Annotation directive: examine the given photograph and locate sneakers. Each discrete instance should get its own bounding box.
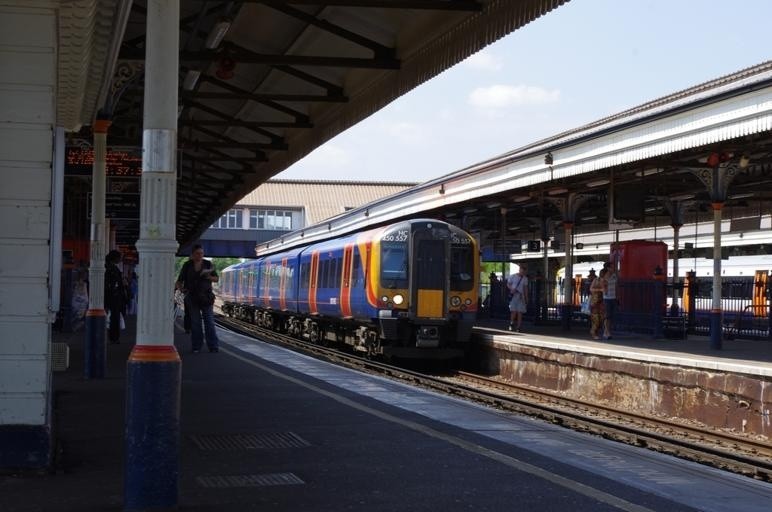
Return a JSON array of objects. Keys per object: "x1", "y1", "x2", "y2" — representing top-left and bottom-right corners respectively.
[
  {"x1": 516, "y1": 328, "x2": 521, "y2": 332},
  {"x1": 209, "y1": 348, "x2": 218, "y2": 353},
  {"x1": 508, "y1": 324, "x2": 512, "y2": 331},
  {"x1": 192, "y1": 349, "x2": 200, "y2": 353},
  {"x1": 592, "y1": 334, "x2": 612, "y2": 340}
]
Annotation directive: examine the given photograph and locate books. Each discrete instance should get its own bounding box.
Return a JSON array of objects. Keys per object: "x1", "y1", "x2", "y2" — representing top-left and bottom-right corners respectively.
[{"x1": 200, "y1": 269, "x2": 213, "y2": 277}]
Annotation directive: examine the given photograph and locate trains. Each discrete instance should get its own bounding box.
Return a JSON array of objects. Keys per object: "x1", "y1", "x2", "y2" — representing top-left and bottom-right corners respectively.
[
  {"x1": 554, "y1": 255, "x2": 771, "y2": 330},
  {"x1": 217, "y1": 218, "x2": 481, "y2": 374}
]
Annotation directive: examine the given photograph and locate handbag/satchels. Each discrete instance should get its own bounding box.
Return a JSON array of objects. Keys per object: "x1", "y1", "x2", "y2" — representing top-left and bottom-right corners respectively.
[
  {"x1": 590, "y1": 292, "x2": 602, "y2": 307},
  {"x1": 192, "y1": 287, "x2": 214, "y2": 306},
  {"x1": 508, "y1": 292, "x2": 513, "y2": 301},
  {"x1": 105, "y1": 312, "x2": 125, "y2": 329}
]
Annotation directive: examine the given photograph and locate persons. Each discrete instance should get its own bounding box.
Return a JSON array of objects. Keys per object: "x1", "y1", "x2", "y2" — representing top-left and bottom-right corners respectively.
[
  {"x1": 175, "y1": 244, "x2": 220, "y2": 355},
  {"x1": 589, "y1": 268, "x2": 610, "y2": 340},
  {"x1": 126, "y1": 271, "x2": 138, "y2": 315},
  {"x1": 506, "y1": 266, "x2": 529, "y2": 332},
  {"x1": 600, "y1": 262, "x2": 622, "y2": 339},
  {"x1": 104, "y1": 250, "x2": 126, "y2": 344}
]
